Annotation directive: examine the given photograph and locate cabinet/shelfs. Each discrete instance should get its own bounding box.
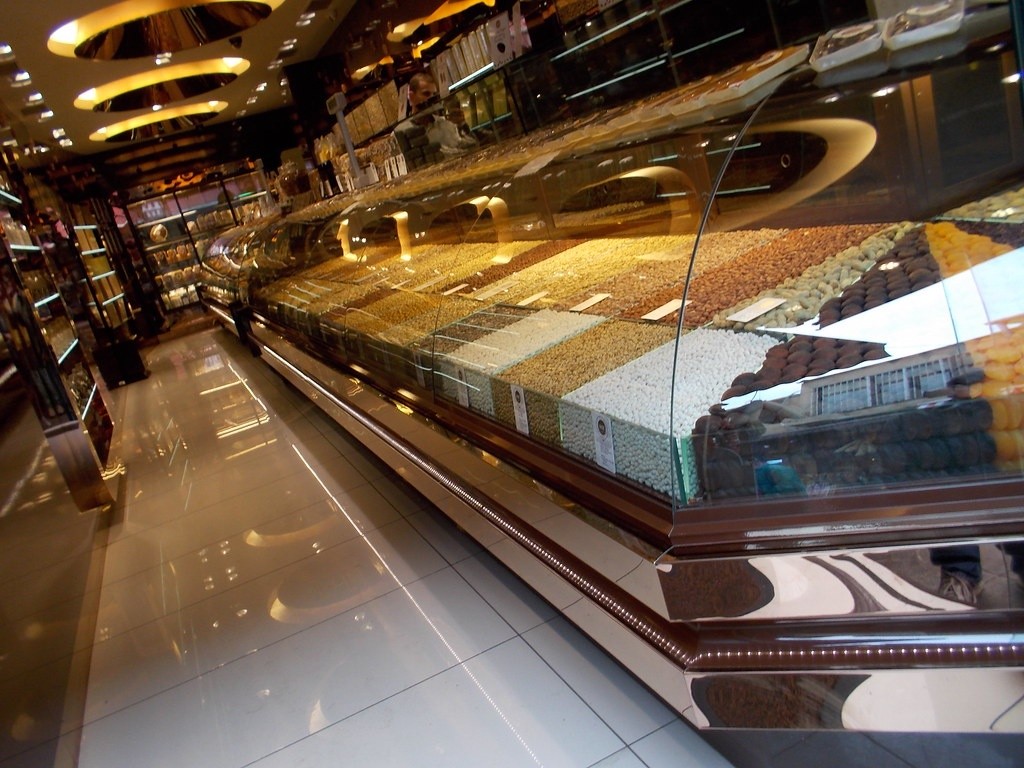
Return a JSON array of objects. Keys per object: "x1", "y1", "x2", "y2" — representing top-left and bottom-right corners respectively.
[{"x1": 0, "y1": 149, "x2": 277, "y2": 513}]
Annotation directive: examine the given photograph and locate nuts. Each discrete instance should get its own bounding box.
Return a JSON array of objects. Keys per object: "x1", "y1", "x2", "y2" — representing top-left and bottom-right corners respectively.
[{"x1": 573, "y1": 221, "x2": 917, "y2": 329}]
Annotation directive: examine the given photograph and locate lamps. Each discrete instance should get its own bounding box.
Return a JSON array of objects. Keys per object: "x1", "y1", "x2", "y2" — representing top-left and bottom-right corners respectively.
[
  {"x1": 73, "y1": 57, "x2": 251, "y2": 113},
  {"x1": 88, "y1": 101, "x2": 228, "y2": 143},
  {"x1": 46, "y1": 0, "x2": 285, "y2": 62}
]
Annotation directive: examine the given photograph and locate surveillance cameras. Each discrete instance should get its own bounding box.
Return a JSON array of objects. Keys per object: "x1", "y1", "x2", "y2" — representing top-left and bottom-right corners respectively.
[{"x1": 229, "y1": 36, "x2": 242, "y2": 48}]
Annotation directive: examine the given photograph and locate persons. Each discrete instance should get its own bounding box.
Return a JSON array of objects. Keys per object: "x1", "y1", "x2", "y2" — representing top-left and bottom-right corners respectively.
[
  {"x1": 391, "y1": 75, "x2": 478, "y2": 156},
  {"x1": 930, "y1": 541, "x2": 1024, "y2": 607}
]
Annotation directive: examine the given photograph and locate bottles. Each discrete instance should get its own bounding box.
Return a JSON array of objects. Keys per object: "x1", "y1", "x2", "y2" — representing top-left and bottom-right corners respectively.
[
  {"x1": 65, "y1": 362, "x2": 94, "y2": 414},
  {"x1": 1, "y1": 216, "x2": 33, "y2": 246},
  {"x1": 315, "y1": 150, "x2": 346, "y2": 200},
  {"x1": 40, "y1": 315, "x2": 75, "y2": 360},
  {"x1": 20, "y1": 269, "x2": 56, "y2": 302}
]
